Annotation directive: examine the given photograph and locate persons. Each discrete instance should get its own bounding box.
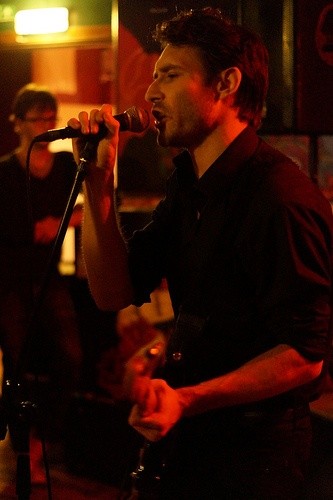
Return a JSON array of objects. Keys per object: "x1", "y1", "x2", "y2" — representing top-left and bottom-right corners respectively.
[
  {"x1": 67, "y1": 6, "x2": 333, "y2": 500},
  {"x1": 0, "y1": 91, "x2": 87, "y2": 408}
]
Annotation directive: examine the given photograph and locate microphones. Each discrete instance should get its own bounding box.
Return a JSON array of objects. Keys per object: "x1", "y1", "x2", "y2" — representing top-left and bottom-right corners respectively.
[{"x1": 33, "y1": 106, "x2": 149, "y2": 142}]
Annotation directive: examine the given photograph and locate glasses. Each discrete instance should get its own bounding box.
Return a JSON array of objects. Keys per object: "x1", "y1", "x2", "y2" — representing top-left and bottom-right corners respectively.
[{"x1": 23, "y1": 116, "x2": 58, "y2": 127}]
